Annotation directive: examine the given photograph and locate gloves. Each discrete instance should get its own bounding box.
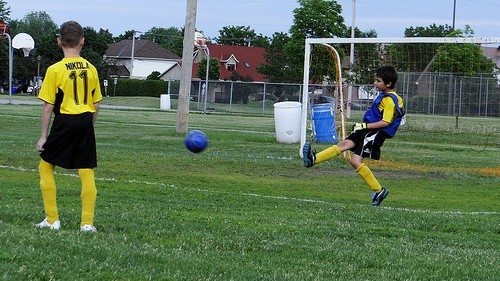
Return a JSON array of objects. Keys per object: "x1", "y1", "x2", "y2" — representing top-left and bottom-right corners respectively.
[{"x1": 352, "y1": 122, "x2": 367, "y2": 133}]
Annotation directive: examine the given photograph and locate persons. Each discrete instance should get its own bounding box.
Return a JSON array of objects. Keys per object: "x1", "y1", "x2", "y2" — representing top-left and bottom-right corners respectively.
[
  {"x1": 34, "y1": 21, "x2": 102, "y2": 231},
  {"x1": 303, "y1": 66, "x2": 406, "y2": 206}
]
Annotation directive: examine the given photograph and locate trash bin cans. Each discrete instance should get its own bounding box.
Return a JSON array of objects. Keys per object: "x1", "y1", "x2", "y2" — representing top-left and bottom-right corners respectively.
[
  {"x1": 273, "y1": 101, "x2": 302, "y2": 143},
  {"x1": 310, "y1": 103, "x2": 338, "y2": 144},
  {"x1": 160, "y1": 94, "x2": 170, "y2": 110}
]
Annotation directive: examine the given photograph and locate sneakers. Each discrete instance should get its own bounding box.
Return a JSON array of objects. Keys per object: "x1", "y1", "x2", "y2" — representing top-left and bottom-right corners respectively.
[
  {"x1": 80, "y1": 224, "x2": 97, "y2": 233},
  {"x1": 35, "y1": 217, "x2": 60, "y2": 230},
  {"x1": 371, "y1": 187, "x2": 389, "y2": 206},
  {"x1": 303, "y1": 142, "x2": 316, "y2": 167}
]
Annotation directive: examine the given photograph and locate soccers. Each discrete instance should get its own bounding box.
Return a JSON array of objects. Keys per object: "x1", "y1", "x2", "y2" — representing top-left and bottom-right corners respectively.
[{"x1": 185, "y1": 130, "x2": 207, "y2": 153}]
